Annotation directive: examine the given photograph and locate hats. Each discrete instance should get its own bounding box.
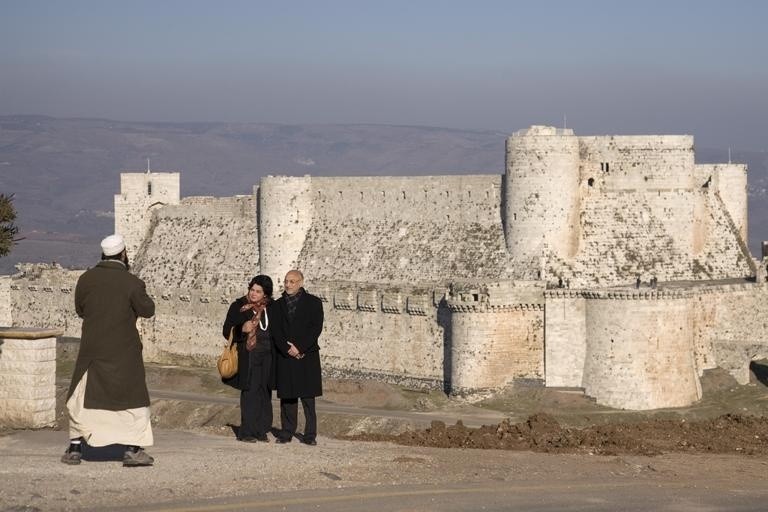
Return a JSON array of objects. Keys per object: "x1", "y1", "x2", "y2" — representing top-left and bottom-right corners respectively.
[{"x1": 100, "y1": 233, "x2": 127, "y2": 258}]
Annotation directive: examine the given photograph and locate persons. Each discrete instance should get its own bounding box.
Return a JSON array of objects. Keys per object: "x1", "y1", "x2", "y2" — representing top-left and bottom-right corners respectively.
[
  {"x1": 269, "y1": 270, "x2": 324, "y2": 446},
  {"x1": 61, "y1": 235, "x2": 156, "y2": 466},
  {"x1": 222, "y1": 274, "x2": 277, "y2": 444}
]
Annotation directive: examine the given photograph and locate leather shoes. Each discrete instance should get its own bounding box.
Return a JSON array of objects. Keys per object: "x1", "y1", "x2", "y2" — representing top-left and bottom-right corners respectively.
[{"x1": 239, "y1": 426, "x2": 319, "y2": 446}]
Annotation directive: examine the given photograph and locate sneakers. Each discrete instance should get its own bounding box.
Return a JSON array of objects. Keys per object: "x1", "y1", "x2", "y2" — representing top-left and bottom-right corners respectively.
[
  {"x1": 121, "y1": 448, "x2": 154, "y2": 466},
  {"x1": 59, "y1": 448, "x2": 83, "y2": 464}
]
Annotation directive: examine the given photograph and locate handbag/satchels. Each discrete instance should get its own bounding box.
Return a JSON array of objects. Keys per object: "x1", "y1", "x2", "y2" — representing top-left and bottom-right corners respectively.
[{"x1": 216, "y1": 322, "x2": 239, "y2": 380}]
